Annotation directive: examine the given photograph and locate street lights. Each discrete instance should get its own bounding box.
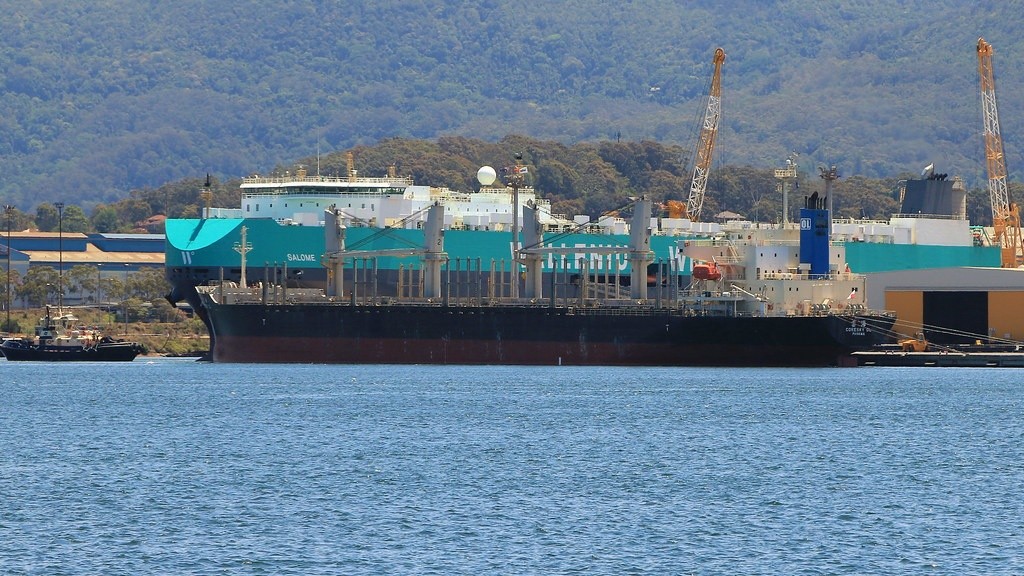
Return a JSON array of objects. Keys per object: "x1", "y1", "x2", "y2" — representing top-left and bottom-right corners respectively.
[
  {"x1": 97, "y1": 263, "x2": 103, "y2": 323},
  {"x1": 55, "y1": 202, "x2": 64, "y2": 315},
  {"x1": 4, "y1": 205, "x2": 14, "y2": 329},
  {"x1": 124, "y1": 264, "x2": 130, "y2": 333}
]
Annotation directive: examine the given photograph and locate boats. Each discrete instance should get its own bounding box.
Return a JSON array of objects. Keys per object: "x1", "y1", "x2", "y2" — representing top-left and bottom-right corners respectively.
[
  {"x1": 165, "y1": 37, "x2": 1023, "y2": 362},
  {"x1": 858, "y1": 341, "x2": 1024, "y2": 368},
  {"x1": 191, "y1": 178, "x2": 900, "y2": 371},
  {"x1": 0, "y1": 304, "x2": 141, "y2": 361}
]
